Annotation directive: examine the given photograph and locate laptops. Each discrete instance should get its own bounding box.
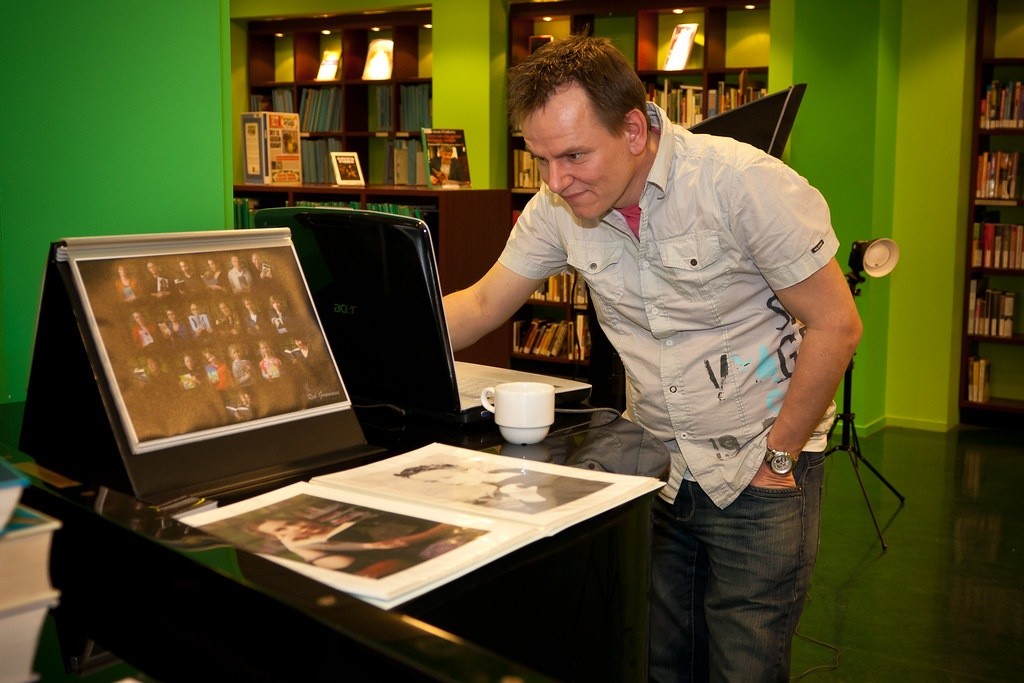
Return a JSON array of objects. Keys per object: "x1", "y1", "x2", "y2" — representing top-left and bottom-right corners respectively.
[{"x1": 254, "y1": 205, "x2": 592, "y2": 432}]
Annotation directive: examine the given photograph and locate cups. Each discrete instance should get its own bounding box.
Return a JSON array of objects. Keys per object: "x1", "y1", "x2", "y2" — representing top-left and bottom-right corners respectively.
[
  {"x1": 499, "y1": 445, "x2": 554, "y2": 464},
  {"x1": 480, "y1": 383, "x2": 555, "y2": 446}
]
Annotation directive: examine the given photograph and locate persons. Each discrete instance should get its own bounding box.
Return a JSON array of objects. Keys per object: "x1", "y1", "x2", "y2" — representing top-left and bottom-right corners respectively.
[
  {"x1": 248, "y1": 512, "x2": 460, "y2": 581},
  {"x1": 115, "y1": 253, "x2": 309, "y2": 421},
  {"x1": 397, "y1": 464, "x2": 613, "y2": 514},
  {"x1": 426, "y1": 146, "x2": 466, "y2": 182},
  {"x1": 440, "y1": 34, "x2": 864, "y2": 682}
]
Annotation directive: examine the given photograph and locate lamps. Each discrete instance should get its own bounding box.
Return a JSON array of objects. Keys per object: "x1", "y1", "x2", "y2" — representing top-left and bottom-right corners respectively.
[{"x1": 820, "y1": 236, "x2": 906, "y2": 555}]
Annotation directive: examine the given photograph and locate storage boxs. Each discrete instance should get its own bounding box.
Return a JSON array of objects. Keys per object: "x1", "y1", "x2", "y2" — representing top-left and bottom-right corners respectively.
[{"x1": 240, "y1": 112, "x2": 304, "y2": 187}]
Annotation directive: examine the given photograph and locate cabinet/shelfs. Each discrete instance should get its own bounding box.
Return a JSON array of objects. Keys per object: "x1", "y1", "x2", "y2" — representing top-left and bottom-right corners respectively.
[
  {"x1": 959, "y1": 55, "x2": 1024, "y2": 430},
  {"x1": 231, "y1": 7, "x2": 439, "y2": 234},
  {"x1": 504, "y1": 0, "x2": 775, "y2": 370}
]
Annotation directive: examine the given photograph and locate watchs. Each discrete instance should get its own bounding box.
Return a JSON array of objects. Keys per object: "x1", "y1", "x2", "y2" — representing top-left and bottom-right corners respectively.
[{"x1": 762, "y1": 447, "x2": 799, "y2": 475}]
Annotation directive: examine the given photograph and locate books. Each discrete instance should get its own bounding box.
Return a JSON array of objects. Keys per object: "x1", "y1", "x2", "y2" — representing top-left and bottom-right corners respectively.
[
  {"x1": 642, "y1": 69, "x2": 769, "y2": 127},
  {"x1": 976, "y1": 150, "x2": 1022, "y2": 200},
  {"x1": 972, "y1": 222, "x2": 1024, "y2": 269},
  {"x1": 967, "y1": 280, "x2": 1017, "y2": 338},
  {"x1": 238, "y1": 82, "x2": 471, "y2": 246},
  {"x1": 968, "y1": 357, "x2": 991, "y2": 403},
  {"x1": 980, "y1": 80, "x2": 1024, "y2": 129},
  {"x1": 509, "y1": 147, "x2": 593, "y2": 362}
]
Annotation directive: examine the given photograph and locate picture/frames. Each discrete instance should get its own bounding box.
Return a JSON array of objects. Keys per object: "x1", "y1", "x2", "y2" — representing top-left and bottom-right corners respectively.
[{"x1": 330, "y1": 152, "x2": 366, "y2": 185}]
